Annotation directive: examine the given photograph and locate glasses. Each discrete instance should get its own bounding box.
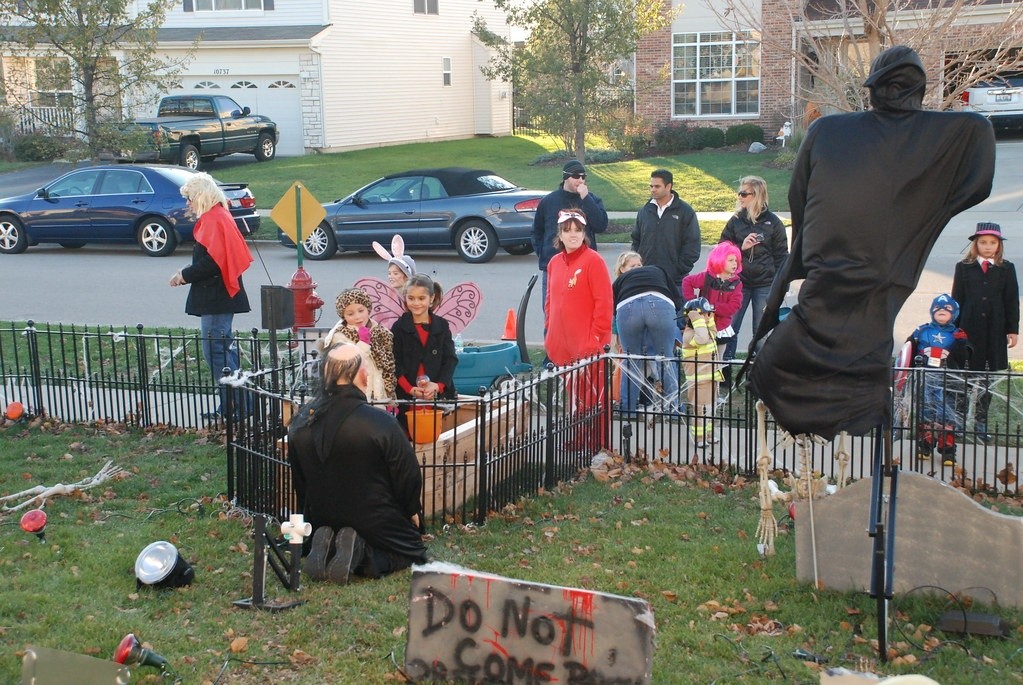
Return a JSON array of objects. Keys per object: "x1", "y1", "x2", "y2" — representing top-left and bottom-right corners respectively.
[
  {"x1": 187, "y1": 195, "x2": 191, "y2": 202},
  {"x1": 738, "y1": 190, "x2": 753, "y2": 198},
  {"x1": 562, "y1": 171, "x2": 587, "y2": 180}
]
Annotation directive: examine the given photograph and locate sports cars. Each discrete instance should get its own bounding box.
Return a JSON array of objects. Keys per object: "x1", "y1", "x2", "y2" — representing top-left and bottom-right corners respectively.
[{"x1": 277, "y1": 167, "x2": 554, "y2": 264}]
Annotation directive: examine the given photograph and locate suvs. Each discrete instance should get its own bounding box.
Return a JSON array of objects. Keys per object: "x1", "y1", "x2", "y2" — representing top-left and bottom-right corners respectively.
[{"x1": 963, "y1": 70, "x2": 1023, "y2": 131}]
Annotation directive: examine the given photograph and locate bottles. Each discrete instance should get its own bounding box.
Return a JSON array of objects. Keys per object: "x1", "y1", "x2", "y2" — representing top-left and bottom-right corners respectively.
[{"x1": 454, "y1": 332, "x2": 463, "y2": 354}]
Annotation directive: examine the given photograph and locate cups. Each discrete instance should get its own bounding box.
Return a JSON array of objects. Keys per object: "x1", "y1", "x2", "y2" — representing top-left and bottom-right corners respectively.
[{"x1": 416, "y1": 375, "x2": 430, "y2": 392}]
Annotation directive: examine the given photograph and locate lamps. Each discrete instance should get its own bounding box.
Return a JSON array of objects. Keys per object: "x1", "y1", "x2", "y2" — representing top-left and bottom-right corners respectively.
[
  {"x1": 21, "y1": 510, "x2": 48, "y2": 545},
  {"x1": 112, "y1": 633, "x2": 167, "y2": 672},
  {"x1": 134, "y1": 540, "x2": 194, "y2": 588}
]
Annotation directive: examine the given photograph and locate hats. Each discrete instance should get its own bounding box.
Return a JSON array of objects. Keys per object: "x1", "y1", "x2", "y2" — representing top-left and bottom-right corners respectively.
[
  {"x1": 562, "y1": 160, "x2": 585, "y2": 180},
  {"x1": 968, "y1": 222, "x2": 1008, "y2": 242},
  {"x1": 335, "y1": 287, "x2": 372, "y2": 320}
]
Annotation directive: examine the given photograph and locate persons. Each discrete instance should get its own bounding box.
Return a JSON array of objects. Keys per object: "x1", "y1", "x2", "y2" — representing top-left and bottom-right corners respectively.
[
  {"x1": 286, "y1": 253, "x2": 459, "y2": 589},
  {"x1": 532, "y1": 159, "x2": 790, "y2": 461},
  {"x1": 892, "y1": 223, "x2": 1020, "y2": 464},
  {"x1": 171, "y1": 170, "x2": 260, "y2": 427}
]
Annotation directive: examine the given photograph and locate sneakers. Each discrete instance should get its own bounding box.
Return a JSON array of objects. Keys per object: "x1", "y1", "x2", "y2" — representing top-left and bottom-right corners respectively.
[
  {"x1": 326, "y1": 526, "x2": 366, "y2": 585},
  {"x1": 917, "y1": 440, "x2": 931, "y2": 459},
  {"x1": 941, "y1": 445, "x2": 956, "y2": 466},
  {"x1": 303, "y1": 525, "x2": 335, "y2": 581}
]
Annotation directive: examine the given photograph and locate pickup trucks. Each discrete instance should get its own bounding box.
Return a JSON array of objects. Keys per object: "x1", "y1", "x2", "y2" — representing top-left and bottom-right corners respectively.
[{"x1": 94, "y1": 95, "x2": 279, "y2": 172}]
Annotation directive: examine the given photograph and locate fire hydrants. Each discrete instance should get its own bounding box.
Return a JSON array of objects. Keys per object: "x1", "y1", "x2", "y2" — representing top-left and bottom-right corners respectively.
[{"x1": 287, "y1": 265, "x2": 324, "y2": 347}]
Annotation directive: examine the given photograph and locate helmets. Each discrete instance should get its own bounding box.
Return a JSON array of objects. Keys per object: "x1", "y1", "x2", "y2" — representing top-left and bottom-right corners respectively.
[{"x1": 683, "y1": 296, "x2": 716, "y2": 317}]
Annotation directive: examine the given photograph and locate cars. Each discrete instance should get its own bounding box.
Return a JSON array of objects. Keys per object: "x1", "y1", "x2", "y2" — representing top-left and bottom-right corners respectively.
[{"x1": 0, "y1": 163, "x2": 260, "y2": 258}]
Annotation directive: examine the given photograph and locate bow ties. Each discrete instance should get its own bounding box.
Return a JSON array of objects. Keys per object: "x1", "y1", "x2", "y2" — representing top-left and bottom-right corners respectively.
[{"x1": 712, "y1": 277, "x2": 731, "y2": 291}]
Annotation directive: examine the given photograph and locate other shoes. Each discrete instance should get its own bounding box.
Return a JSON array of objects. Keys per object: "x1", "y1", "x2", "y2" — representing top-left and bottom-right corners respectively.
[
  {"x1": 225, "y1": 413, "x2": 253, "y2": 424},
  {"x1": 576, "y1": 448, "x2": 598, "y2": 458},
  {"x1": 707, "y1": 437, "x2": 720, "y2": 444},
  {"x1": 556, "y1": 439, "x2": 586, "y2": 451},
  {"x1": 693, "y1": 440, "x2": 709, "y2": 448},
  {"x1": 199, "y1": 410, "x2": 220, "y2": 419},
  {"x1": 955, "y1": 418, "x2": 966, "y2": 438},
  {"x1": 973, "y1": 420, "x2": 992, "y2": 441}
]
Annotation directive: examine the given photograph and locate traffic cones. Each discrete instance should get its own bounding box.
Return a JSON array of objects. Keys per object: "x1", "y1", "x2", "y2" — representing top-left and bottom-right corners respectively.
[
  {"x1": 501, "y1": 308, "x2": 517, "y2": 340},
  {"x1": 612, "y1": 349, "x2": 623, "y2": 402}
]
